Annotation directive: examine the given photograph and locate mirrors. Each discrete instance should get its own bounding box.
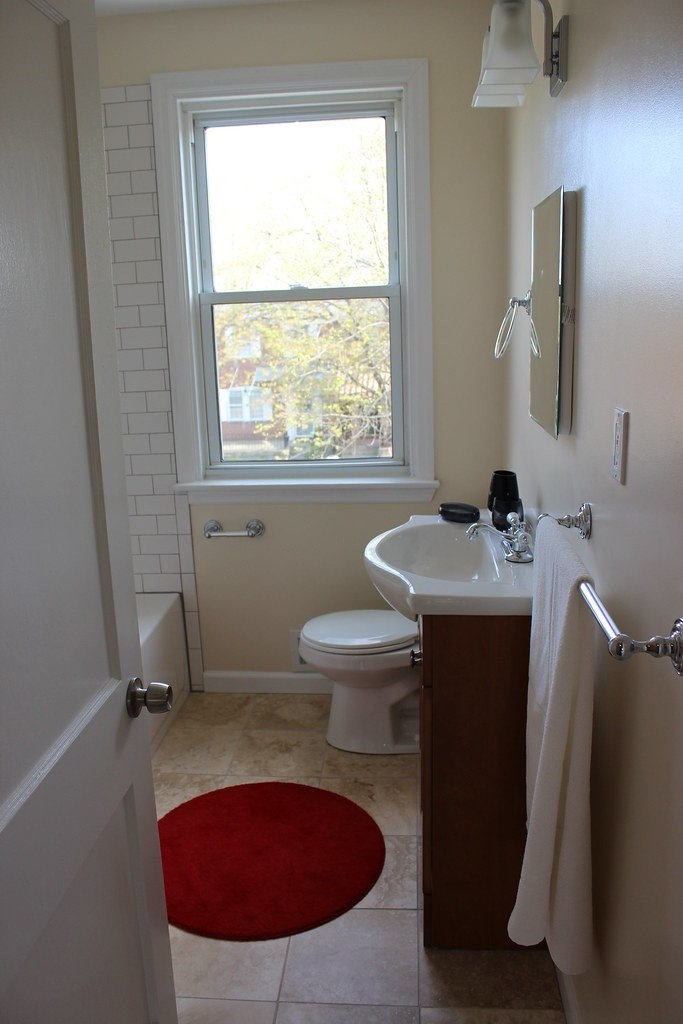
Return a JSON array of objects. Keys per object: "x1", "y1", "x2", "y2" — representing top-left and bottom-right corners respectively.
[{"x1": 524, "y1": 185, "x2": 577, "y2": 441}]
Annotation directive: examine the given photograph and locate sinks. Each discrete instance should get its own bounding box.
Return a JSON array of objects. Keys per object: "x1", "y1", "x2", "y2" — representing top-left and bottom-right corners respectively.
[{"x1": 362, "y1": 506, "x2": 533, "y2": 623}]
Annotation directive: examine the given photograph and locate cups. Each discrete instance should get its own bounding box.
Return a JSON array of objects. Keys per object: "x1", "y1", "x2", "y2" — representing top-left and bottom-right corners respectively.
[
  {"x1": 487, "y1": 470, "x2": 519, "y2": 512},
  {"x1": 493, "y1": 495, "x2": 523, "y2": 533}
]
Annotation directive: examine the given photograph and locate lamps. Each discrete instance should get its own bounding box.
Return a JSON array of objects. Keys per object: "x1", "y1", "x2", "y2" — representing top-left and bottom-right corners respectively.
[{"x1": 471, "y1": 0, "x2": 569, "y2": 108}]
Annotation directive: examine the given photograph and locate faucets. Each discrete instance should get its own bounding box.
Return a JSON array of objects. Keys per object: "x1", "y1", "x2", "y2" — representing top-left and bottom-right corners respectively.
[{"x1": 464, "y1": 522, "x2": 515, "y2": 542}]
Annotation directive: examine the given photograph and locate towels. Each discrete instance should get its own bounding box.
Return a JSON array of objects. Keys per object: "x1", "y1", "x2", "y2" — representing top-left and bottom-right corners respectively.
[{"x1": 505, "y1": 518, "x2": 601, "y2": 980}]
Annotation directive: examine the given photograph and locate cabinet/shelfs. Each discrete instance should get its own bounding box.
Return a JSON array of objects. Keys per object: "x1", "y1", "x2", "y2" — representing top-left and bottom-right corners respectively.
[{"x1": 407, "y1": 613, "x2": 547, "y2": 955}]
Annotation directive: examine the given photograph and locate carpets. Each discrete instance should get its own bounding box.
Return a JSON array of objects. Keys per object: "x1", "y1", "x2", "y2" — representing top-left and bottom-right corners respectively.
[{"x1": 153, "y1": 781, "x2": 387, "y2": 941}]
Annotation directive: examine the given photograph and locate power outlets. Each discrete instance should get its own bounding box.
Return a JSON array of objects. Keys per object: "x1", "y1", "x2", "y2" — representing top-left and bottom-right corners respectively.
[{"x1": 609, "y1": 406, "x2": 630, "y2": 486}]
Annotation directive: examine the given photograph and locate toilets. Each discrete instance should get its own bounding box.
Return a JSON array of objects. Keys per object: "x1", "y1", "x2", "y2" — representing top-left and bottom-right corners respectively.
[{"x1": 297, "y1": 609, "x2": 421, "y2": 754}]
